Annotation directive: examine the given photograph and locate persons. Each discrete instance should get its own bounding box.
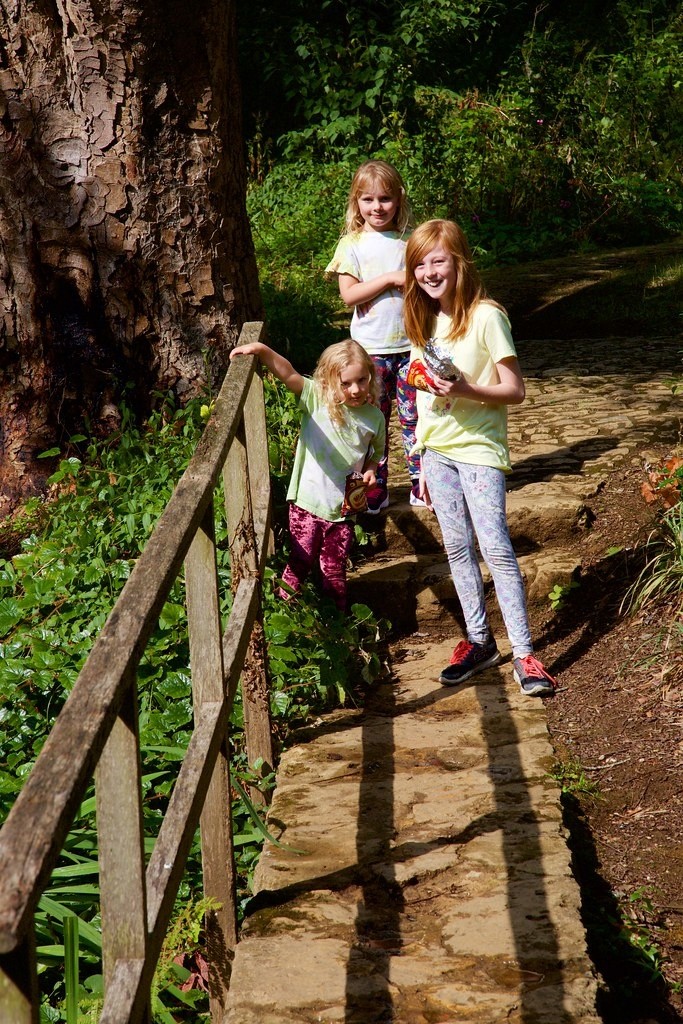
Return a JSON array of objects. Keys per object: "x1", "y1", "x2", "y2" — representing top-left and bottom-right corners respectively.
[
  {"x1": 229, "y1": 339, "x2": 385, "y2": 608},
  {"x1": 323, "y1": 161, "x2": 432, "y2": 509},
  {"x1": 403, "y1": 217, "x2": 553, "y2": 697}
]
